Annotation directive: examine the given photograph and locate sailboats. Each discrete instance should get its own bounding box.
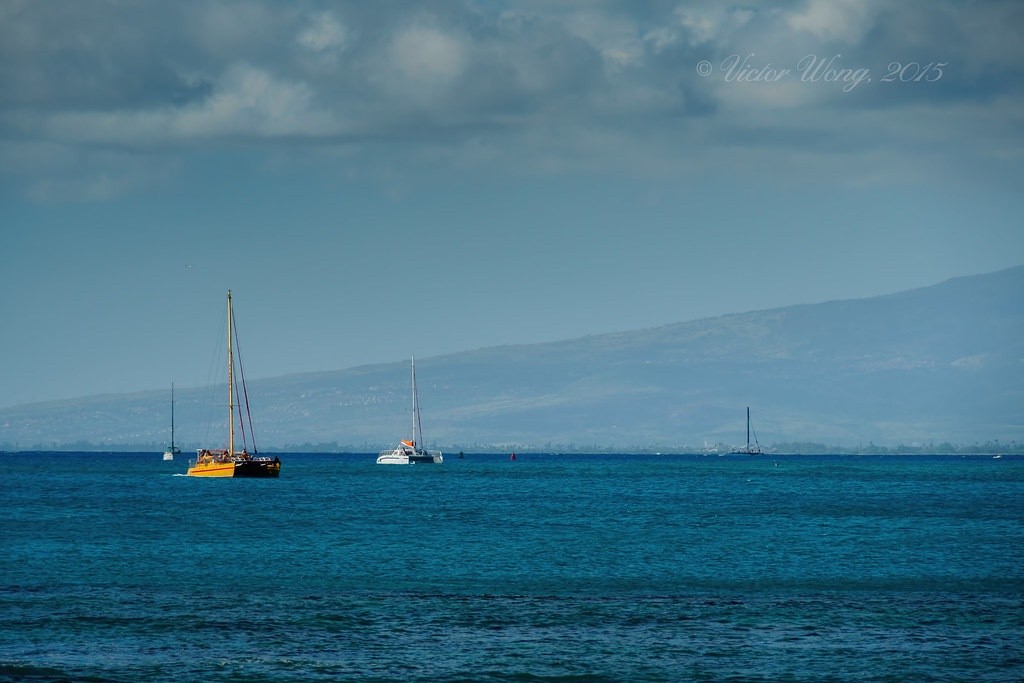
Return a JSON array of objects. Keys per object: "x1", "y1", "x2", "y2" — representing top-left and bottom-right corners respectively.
[
  {"x1": 376, "y1": 354, "x2": 444, "y2": 465},
  {"x1": 186, "y1": 287, "x2": 282, "y2": 478},
  {"x1": 728, "y1": 405, "x2": 765, "y2": 455},
  {"x1": 162, "y1": 379, "x2": 183, "y2": 462}
]
